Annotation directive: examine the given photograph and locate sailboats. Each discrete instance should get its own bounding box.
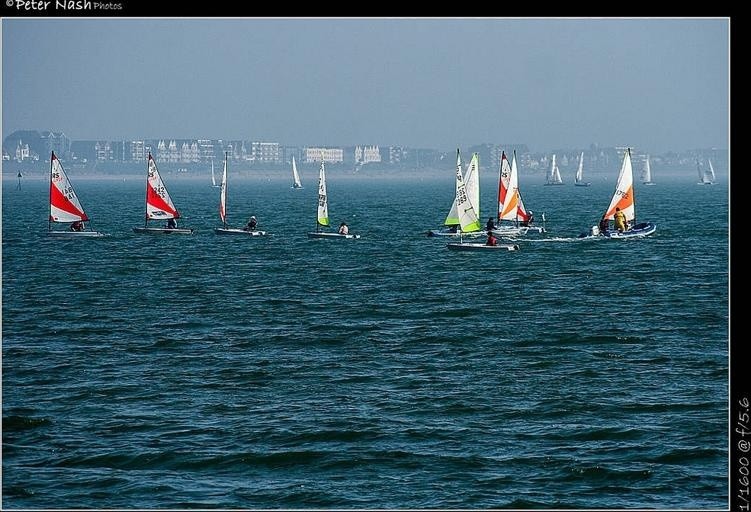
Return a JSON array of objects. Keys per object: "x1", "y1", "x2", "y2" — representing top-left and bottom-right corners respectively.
[
  {"x1": 49, "y1": 150, "x2": 110, "y2": 239},
  {"x1": 696, "y1": 159, "x2": 719, "y2": 187},
  {"x1": 290, "y1": 156, "x2": 305, "y2": 190},
  {"x1": 215, "y1": 150, "x2": 268, "y2": 237},
  {"x1": 429, "y1": 148, "x2": 658, "y2": 252},
  {"x1": 210, "y1": 160, "x2": 220, "y2": 189},
  {"x1": 132, "y1": 152, "x2": 194, "y2": 237},
  {"x1": 306, "y1": 160, "x2": 361, "y2": 241}
]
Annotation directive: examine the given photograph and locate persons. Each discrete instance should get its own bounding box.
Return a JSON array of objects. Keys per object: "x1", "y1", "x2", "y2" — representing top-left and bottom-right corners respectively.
[
  {"x1": 71, "y1": 220, "x2": 85, "y2": 231},
  {"x1": 168, "y1": 218, "x2": 178, "y2": 229},
  {"x1": 601, "y1": 219, "x2": 610, "y2": 232},
  {"x1": 248, "y1": 215, "x2": 257, "y2": 230},
  {"x1": 339, "y1": 223, "x2": 349, "y2": 234},
  {"x1": 487, "y1": 216, "x2": 498, "y2": 231},
  {"x1": 486, "y1": 231, "x2": 497, "y2": 246},
  {"x1": 615, "y1": 207, "x2": 627, "y2": 232},
  {"x1": 528, "y1": 210, "x2": 534, "y2": 225}
]
